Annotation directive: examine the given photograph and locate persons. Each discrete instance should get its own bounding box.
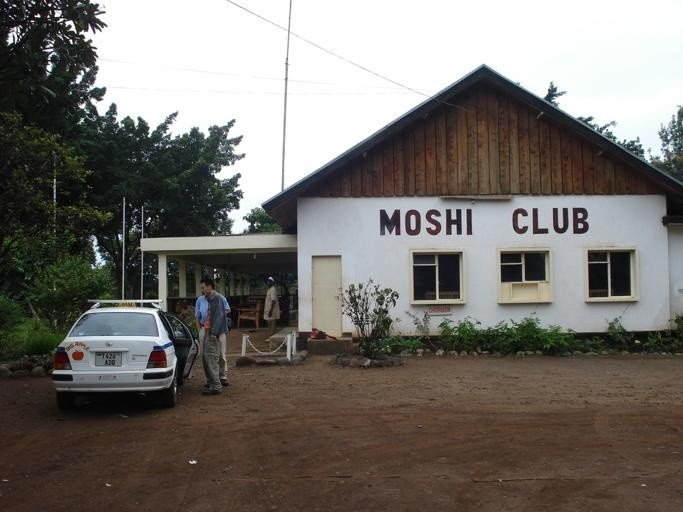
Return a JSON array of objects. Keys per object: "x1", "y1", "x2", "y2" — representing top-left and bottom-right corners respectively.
[
  {"x1": 199, "y1": 278, "x2": 227, "y2": 396},
  {"x1": 195, "y1": 291, "x2": 232, "y2": 387},
  {"x1": 263, "y1": 276, "x2": 281, "y2": 343}
]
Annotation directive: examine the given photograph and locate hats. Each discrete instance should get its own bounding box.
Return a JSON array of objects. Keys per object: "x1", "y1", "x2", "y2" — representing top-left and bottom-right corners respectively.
[{"x1": 268, "y1": 277, "x2": 274, "y2": 283}]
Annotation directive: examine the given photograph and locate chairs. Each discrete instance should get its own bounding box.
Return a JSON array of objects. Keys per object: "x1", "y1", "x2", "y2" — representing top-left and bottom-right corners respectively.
[{"x1": 237, "y1": 300, "x2": 262, "y2": 328}]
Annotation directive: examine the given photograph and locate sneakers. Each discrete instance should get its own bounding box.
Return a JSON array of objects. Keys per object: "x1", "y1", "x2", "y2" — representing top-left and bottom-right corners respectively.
[{"x1": 201, "y1": 379, "x2": 231, "y2": 396}]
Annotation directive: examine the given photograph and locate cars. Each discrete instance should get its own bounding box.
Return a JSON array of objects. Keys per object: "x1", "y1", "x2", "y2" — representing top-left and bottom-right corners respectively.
[{"x1": 51, "y1": 298, "x2": 198, "y2": 411}]
